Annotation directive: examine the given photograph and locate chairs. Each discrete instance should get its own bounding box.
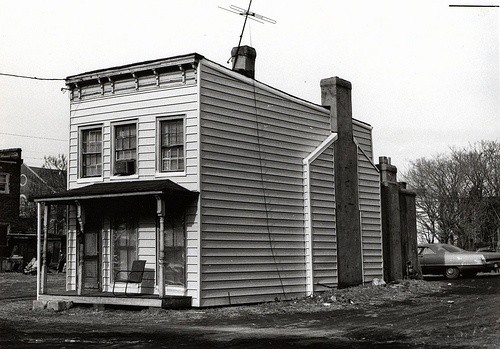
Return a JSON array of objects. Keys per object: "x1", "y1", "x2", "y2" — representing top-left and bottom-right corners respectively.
[{"x1": 112, "y1": 259, "x2": 146, "y2": 297}]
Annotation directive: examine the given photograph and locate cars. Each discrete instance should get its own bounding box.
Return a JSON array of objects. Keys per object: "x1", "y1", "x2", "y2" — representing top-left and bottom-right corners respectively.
[{"x1": 416, "y1": 243, "x2": 500, "y2": 281}]
